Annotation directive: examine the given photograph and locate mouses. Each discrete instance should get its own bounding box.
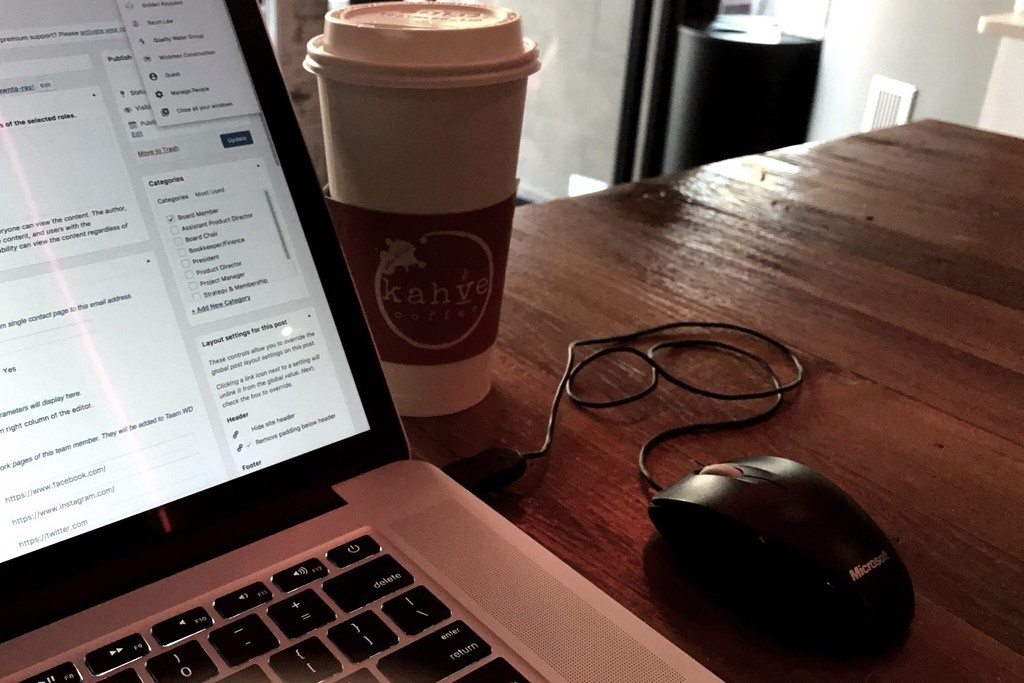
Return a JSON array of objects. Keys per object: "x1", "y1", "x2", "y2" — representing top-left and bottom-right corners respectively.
[{"x1": 649, "y1": 455, "x2": 917, "y2": 664}]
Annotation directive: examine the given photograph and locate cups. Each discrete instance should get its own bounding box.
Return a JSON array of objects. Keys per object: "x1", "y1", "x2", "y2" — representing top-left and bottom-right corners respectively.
[{"x1": 303, "y1": 2, "x2": 542, "y2": 417}]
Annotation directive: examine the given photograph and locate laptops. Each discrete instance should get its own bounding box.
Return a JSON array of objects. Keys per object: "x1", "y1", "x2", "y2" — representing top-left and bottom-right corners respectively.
[{"x1": 0, "y1": 0, "x2": 726, "y2": 683}]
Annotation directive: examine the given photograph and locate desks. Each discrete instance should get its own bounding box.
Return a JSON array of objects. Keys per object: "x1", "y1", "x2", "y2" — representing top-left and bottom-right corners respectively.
[{"x1": 401, "y1": 119, "x2": 1024, "y2": 683}]
